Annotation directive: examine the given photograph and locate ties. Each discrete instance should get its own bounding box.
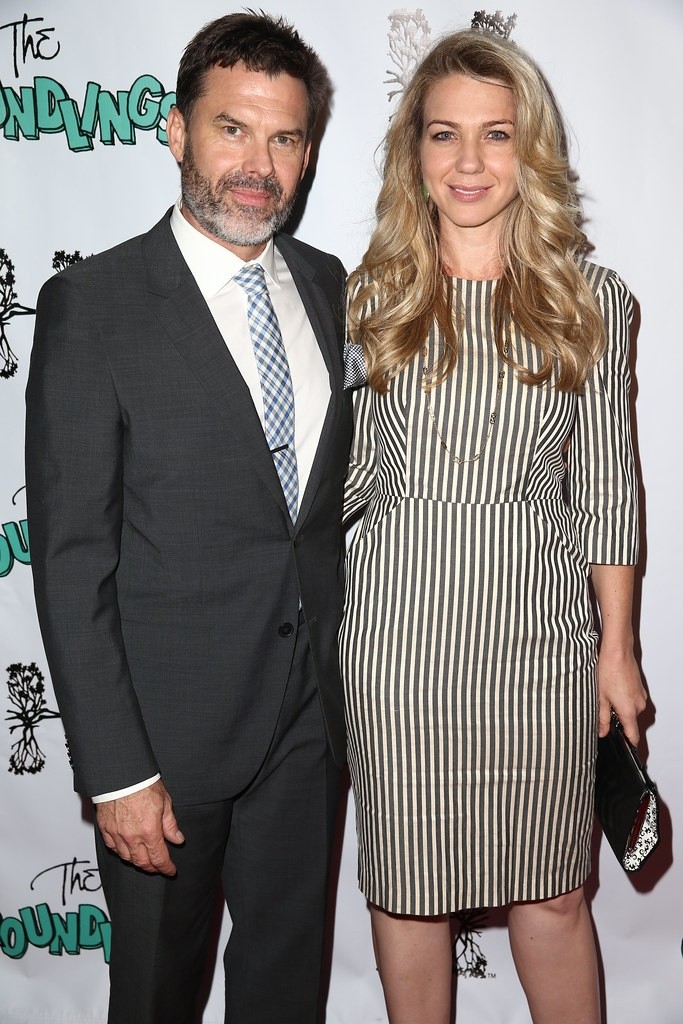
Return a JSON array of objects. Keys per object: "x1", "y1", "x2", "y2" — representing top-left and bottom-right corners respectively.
[{"x1": 233, "y1": 264, "x2": 299, "y2": 527}]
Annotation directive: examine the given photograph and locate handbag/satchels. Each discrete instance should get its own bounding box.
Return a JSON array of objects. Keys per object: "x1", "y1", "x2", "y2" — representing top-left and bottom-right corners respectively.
[{"x1": 593, "y1": 711, "x2": 662, "y2": 875}]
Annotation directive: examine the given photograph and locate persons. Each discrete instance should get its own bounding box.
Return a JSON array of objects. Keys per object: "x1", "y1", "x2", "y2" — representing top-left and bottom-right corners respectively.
[
  {"x1": 341, "y1": 29, "x2": 648, "y2": 1024},
  {"x1": 24, "y1": 11, "x2": 356, "y2": 1024}
]
